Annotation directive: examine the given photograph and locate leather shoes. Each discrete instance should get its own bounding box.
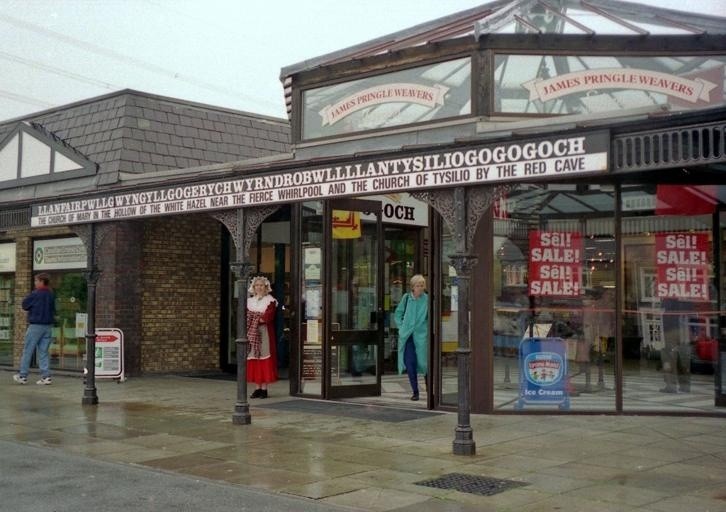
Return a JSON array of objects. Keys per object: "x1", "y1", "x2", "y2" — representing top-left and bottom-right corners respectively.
[
  {"x1": 261, "y1": 389, "x2": 267, "y2": 399},
  {"x1": 411, "y1": 395, "x2": 419, "y2": 400},
  {"x1": 249, "y1": 389, "x2": 261, "y2": 398}
]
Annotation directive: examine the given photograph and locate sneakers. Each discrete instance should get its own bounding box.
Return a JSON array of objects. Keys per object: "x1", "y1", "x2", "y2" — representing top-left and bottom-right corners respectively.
[
  {"x1": 35, "y1": 378, "x2": 52, "y2": 385},
  {"x1": 12, "y1": 374, "x2": 26, "y2": 384}
]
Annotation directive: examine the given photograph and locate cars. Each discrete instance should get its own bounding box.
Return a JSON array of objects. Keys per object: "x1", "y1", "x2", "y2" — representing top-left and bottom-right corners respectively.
[
  {"x1": 658, "y1": 295, "x2": 719, "y2": 312},
  {"x1": 502, "y1": 282, "x2": 602, "y2": 308},
  {"x1": 515, "y1": 297, "x2": 611, "y2": 318}
]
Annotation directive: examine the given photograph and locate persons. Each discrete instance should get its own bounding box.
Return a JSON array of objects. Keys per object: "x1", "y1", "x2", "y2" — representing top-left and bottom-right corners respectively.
[
  {"x1": 246, "y1": 276, "x2": 278, "y2": 399},
  {"x1": 393, "y1": 274, "x2": 432, "y2": 401},
  {"x1": 12, "y1": 272, "x2": 54, "y2": 386},
  {"x1": 653, "y1": 286, "x2": 696, "y2": 396}
]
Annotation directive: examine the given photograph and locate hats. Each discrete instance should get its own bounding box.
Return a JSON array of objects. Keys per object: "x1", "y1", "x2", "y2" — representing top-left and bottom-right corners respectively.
[{"x1": 247, "y1": 275, "x2": 272, "y2": 296}]
